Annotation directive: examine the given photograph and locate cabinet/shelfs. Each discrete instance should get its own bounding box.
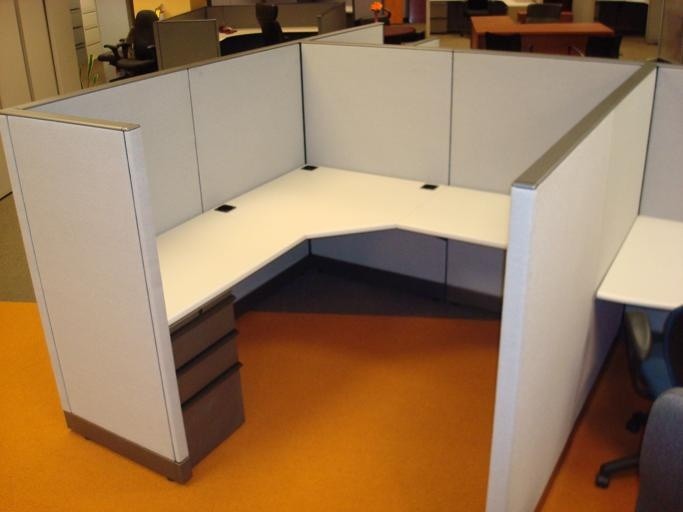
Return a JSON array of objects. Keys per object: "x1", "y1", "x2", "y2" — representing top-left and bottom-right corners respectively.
[
  {"x1": 165, "y1": 288, "x2": 249, "y2": 468},
  {"x1": 595, "y1": 215, "x2": 683, "y2": 312}
]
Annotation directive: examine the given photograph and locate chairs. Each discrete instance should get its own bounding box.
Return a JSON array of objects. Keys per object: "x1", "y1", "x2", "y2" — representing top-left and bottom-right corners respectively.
[
  {"x1": 103, "y1": 38, "x2": 158, "y2": 81},
  {"x1": 254, "y1": 0, "x2": 286, "y2": 48},
  {"x1": 592, "y1": 297, "x2": 681, "y2": 511}
]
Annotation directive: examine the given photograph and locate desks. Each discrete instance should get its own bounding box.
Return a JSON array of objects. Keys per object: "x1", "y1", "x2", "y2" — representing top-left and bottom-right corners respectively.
[
  {"x1": 154, "y1": 158, "x2": 516, "y2": 328},
  {"x1": 216, "y1": 20, "x2": 321, "y2": 44},
  {"x1": 466, "y1": 12, "x2": 612, "y2": 56},
  {"x1": 383, "y1": 25, "x2": 416, "y2": 44}
]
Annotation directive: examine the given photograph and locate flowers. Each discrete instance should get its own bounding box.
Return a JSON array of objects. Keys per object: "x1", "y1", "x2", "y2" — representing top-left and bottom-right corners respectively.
[{"x1": 370, "y1": 0, "x2": 383, "y2": 13}]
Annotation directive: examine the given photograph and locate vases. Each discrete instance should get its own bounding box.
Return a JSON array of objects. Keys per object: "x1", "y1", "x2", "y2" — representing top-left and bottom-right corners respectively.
[{"x1": 374, "y1": 11, "x2": 380, "y2": 22}]
